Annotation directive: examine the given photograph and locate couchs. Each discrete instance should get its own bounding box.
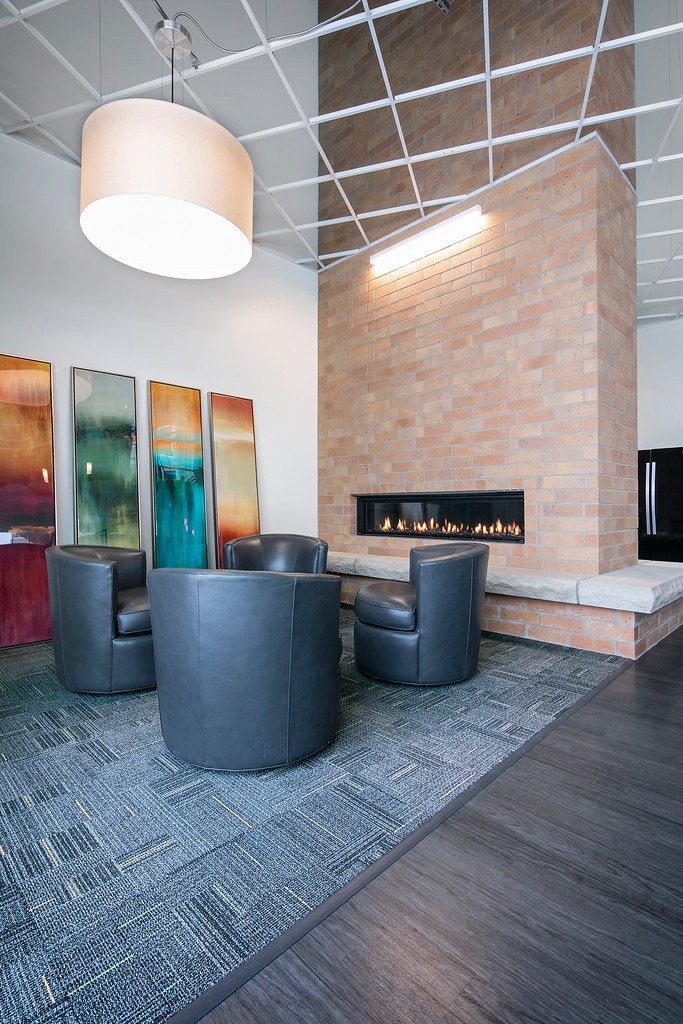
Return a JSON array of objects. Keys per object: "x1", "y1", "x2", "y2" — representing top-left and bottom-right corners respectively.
[
  {"x1": 224, "y1": 533, "x2": 329, "y2": 573},
  {"x1": 146, "y1": 568, "x2": 343, "y2": 771},
  {"x1": 353, "y1": 542, "x2": 489, "y2": 686},
  {"x1": 45, "y1": 544, "x2": 157, "y2": 695}
]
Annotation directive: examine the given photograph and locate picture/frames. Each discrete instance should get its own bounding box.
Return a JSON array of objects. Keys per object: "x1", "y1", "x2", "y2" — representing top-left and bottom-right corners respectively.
[
  {"x1": 69, "y1": 365, "x2": 142, "y2": 549},
  {"x1": 207, "y1": 391, "x2": 260, "y2": 569},
  {"x1": 0, "y1": 353, "x2": 58, "y2": 546},
  {"x1": 146, "y1": 380, "x2": 209, "y2": 569}
]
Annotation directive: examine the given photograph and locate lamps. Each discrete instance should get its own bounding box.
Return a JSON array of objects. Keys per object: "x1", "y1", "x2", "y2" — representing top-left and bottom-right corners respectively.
[
  {"x1": 369, "y1": 204, "x2": 484, "y2": 268},
  {"x1": 80, "y1": 20, "x2": 254, "y2": 280}
]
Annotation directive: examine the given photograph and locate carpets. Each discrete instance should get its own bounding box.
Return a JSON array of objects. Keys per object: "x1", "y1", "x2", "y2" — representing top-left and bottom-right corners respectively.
[{"x1": 0, "y1": 606, "x2": 634, "y2": 1024}]
